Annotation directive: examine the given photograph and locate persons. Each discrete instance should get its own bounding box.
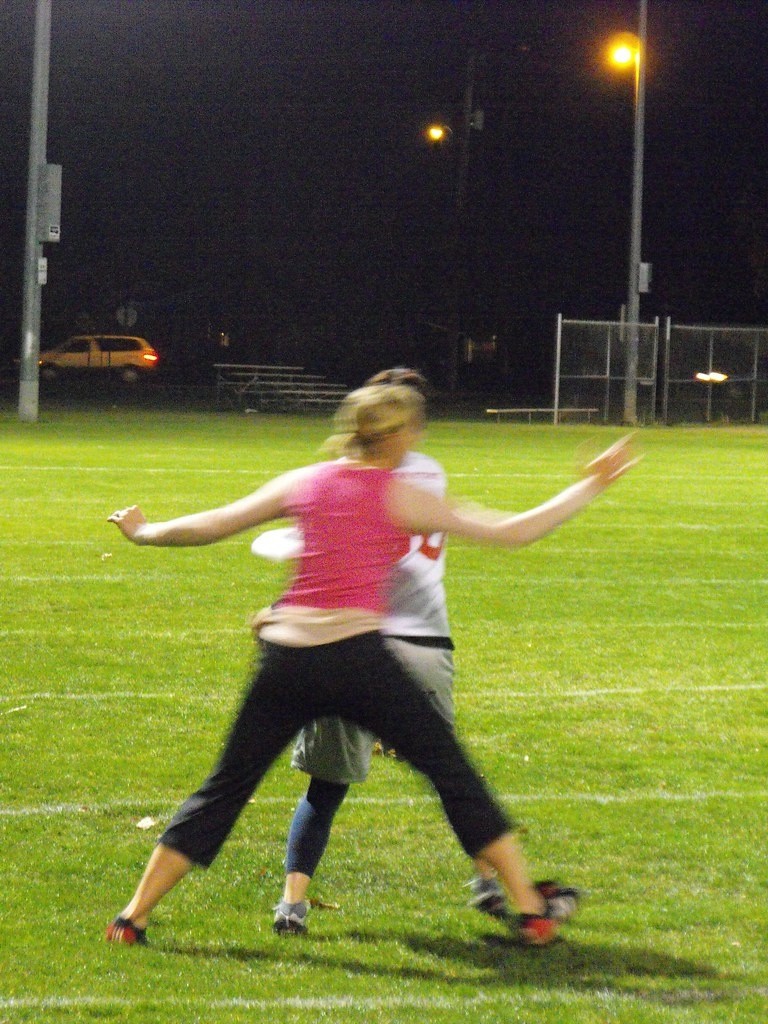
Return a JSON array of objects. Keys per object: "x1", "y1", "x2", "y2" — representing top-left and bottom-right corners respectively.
[{"x1": 105, "y1": 367, "x2": 642, "y2": 949}]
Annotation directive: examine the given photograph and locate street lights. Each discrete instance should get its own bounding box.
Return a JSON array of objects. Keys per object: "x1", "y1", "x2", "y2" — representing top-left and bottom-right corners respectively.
[
  {"x1": 604, "y1": 0, "x2": 649, "y2": 429},
  {"x1": 420, "y1": 53, "x2": 481, "y2": 394}
]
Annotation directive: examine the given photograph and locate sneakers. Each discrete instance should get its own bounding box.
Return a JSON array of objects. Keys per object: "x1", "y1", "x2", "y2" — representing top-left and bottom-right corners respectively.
[
  {"x1": 469, "y1": 880, "x2": 517, "y2": 920},
  {"x1": 271, "y1": 900, "x2": 308, "y2": 934},
  {"x1": 516, "y1": 882, "x2": 580, "y2": 947},
  {"x1": 106, "y1": 917, "x2": 147, "y2": 944}
]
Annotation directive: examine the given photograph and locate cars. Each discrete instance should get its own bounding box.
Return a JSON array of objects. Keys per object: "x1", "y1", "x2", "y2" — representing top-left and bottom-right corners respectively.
[{"x1": 13, "y1": 334, "x2": 168, "y2": 386}]
